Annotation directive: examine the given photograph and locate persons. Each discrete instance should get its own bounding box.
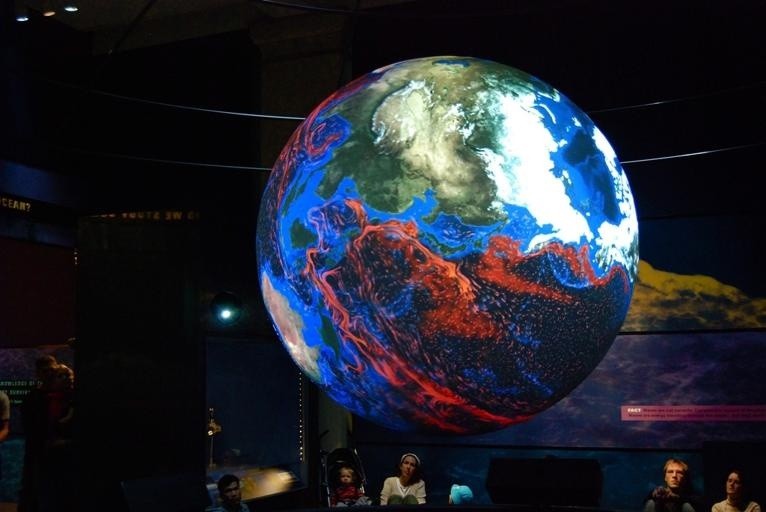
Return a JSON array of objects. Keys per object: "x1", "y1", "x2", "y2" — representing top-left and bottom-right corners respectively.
[
  {"x1": 709, "y1": 467, "x2": 765, "y2": 512},
  {"x1": 447, "y1": 483, "x2": 473, "y2": 504},
  {"x1": 34, "y1": 351, "x2": 83, "y2": 429},
  {"x1": 641, "y1": 455, "x2": 700, "y2": 511},
  {"x1": 0, "y1": 389, "x2": 14, "y2": 446},
  {"x1": 325, "y1": 463, "x2": 368, "y2": 512},
  {"x1": 206, "y1": 473, "x2": 256, "y2": 512},
  {"x1": 377, "y1": 450, "x2": 429, "y2": 508}
]
